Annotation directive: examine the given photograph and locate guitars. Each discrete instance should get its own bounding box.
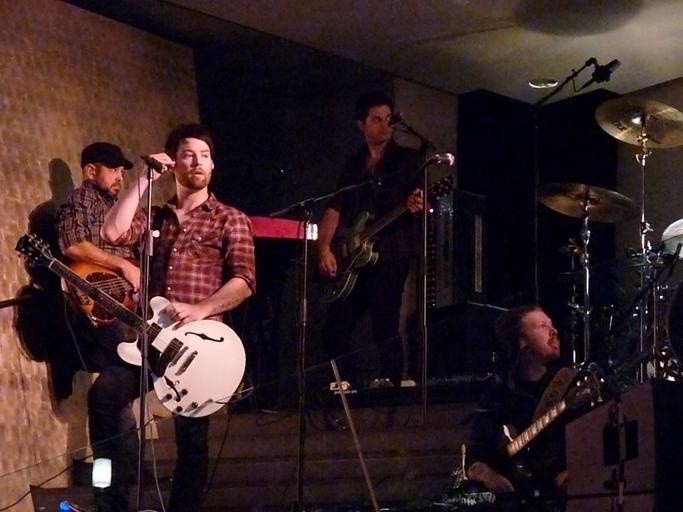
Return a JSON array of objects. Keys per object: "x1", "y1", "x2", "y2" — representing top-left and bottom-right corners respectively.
[
  {"x1": 58, "y1": 256, "x2": 140, "y2": 327},
  {"x1": 16, "y1": 232, "x2": 245, "y2": 418},
  {"x1": 316, "y1": 175, "x2": 456, "y2": 305},
  {"x1": 437, "y1": 370, "x2": 602, "y2": 511}
]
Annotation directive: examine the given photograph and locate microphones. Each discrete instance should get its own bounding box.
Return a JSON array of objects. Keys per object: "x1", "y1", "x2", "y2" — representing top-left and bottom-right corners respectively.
[
  {"x1": 141, "y1": 152, "x2": 169, "y2": 176},
  {"x1": 357, "y1": 173, "x2": 383, "y2": 192},
  {"x1": 388, "y1": 111, "x2": 405, "y2": 130},
  {"x1": 583, "y1": 58, "x2": 621, "y2": 89},
  {"x1": 426, "y1": 151, "x2": 456, "y2": 168}
]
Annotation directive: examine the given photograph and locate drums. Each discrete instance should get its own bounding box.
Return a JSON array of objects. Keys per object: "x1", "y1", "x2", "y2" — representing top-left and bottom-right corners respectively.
[{"x1": 666, "y1": 279, "x2": 682, "y2": 369}]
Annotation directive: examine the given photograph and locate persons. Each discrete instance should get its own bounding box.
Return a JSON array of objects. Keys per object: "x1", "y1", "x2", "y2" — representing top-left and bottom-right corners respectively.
[
  {"x1": 87, "y1": 122, "x2": 259, "y2": 512},
  {"x1": 54, "y1": 143, "x2": 160, "y2": 488},
  {"x1": 455, "y1": 304, "x2": 599, "y2": 511},
  {"x1": 315, "y1": 94, "x2": 433, "y2": 394}
]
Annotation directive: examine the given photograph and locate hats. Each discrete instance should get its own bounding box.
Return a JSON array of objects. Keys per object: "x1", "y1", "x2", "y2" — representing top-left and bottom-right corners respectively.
[{"x1": 82, "y1": 142, "x2": 133, "y2": 169}]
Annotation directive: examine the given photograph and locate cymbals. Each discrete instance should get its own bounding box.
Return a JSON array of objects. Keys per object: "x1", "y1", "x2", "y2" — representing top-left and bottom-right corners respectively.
[
  {"x1": 594, "y1": 96, "x2": 682, "y2": 149},
  {"x1": 535, "y1": 179, "x2": 636, "y2": 224}
]
